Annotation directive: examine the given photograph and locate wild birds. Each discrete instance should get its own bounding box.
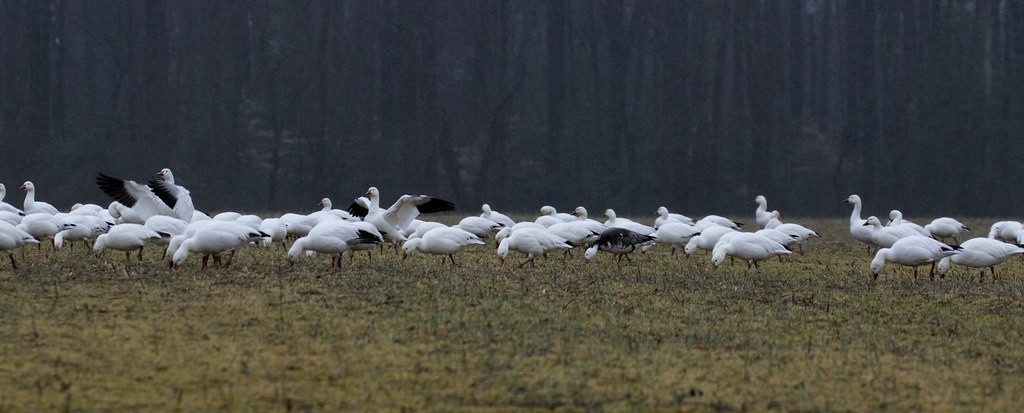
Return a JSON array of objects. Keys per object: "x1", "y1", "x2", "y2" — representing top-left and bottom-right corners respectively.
[
  {"x1": 0, "y1": 168, "x2": 820, "y2": 270},
  {"x1": 841, "y1": 194, "x2": 1024, "y2": 283}
]
